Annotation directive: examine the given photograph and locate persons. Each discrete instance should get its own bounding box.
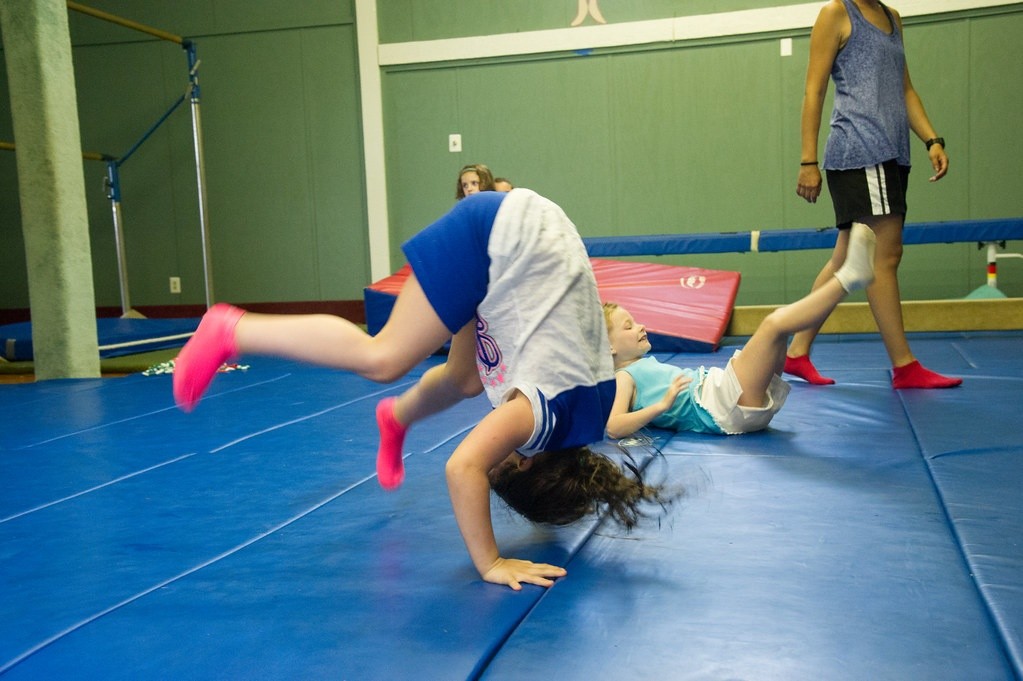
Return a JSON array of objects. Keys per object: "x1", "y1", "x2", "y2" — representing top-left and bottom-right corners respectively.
[
  {"x1": 456, "y1": 165, "x2": 493, "y2": 199},
  {"x1": 175, "y1": 188, "x2": 654, "y2": 590},
  {"x1": 784, "y1": 1, "x2": 965, "y2": 389},
  {"x1": 604, "y1": 221, "x2": 877, "y2": 440},
  {"x1": 493, "y1": 178, "x2": 514, "y2": 192}
]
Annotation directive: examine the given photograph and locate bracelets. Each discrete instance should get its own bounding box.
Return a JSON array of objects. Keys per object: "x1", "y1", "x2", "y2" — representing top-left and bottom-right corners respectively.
[
  {"x1": 927, "y1": 137, "x2": 945, "y2": 152},
  {"x1": 801, "y1": 161, "x2": 818, "y2": 165}
]
